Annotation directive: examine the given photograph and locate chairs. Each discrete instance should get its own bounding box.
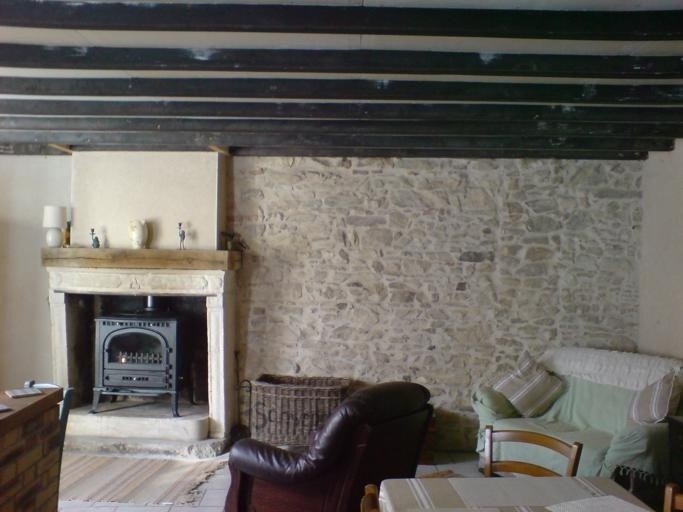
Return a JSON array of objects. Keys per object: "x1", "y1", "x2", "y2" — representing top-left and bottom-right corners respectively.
[
  {"x1": 482, "y1": 425, "x2": 582, "y2": 478},
  {"x1": 224, "y1": 380, "x2": 434, "y2": 510}
]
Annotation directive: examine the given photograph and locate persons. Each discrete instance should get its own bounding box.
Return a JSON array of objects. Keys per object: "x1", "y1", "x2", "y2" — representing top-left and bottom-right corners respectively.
[{"x1": 178, "y1": 223, "x2": 185, "y2": 249}]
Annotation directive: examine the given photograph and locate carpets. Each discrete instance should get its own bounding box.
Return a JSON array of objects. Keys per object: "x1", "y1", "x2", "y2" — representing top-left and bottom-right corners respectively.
[{"x1": 59, "y1": 455, "x2": 228, "y2": 506}]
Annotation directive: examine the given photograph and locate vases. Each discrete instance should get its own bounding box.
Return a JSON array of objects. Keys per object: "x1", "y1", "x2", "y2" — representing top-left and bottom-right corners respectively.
[{"x1": 129, "y1": 220, "x2": 149, "y2": 249}]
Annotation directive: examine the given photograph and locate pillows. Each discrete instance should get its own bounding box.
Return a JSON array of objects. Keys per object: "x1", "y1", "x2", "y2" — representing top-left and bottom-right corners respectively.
[
  {"x1": 496, "y1": 349, "x2": 566, "y2": 420},
  {"x1": 624, "y1": 372, "x2": 675, "y2": 425}
]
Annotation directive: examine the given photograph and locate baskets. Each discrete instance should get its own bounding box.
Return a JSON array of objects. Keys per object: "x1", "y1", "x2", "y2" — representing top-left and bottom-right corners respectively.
[{"x1": 250, "y1": 373, "x2": 352, "y2": 447}]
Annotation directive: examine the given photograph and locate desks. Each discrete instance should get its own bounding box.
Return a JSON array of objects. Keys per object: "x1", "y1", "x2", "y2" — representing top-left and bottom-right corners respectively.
[
  {"x1": 379, "y1": 478, "x2": 659, "y2": 512},
  {"x1": 0, "y1": 388, "x2": 66, "y2": 511}
]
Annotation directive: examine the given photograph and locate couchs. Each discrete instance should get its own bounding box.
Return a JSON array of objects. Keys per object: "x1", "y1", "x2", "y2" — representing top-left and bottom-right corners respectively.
[{"x1": 470, "y1": 346, "x2": 683, "y2": 477}]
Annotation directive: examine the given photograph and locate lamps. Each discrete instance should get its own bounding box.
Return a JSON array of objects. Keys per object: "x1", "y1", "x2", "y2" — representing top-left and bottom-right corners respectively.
[{"x1": 43, "y1": 206, "x2": 68, "y2": 248}]
